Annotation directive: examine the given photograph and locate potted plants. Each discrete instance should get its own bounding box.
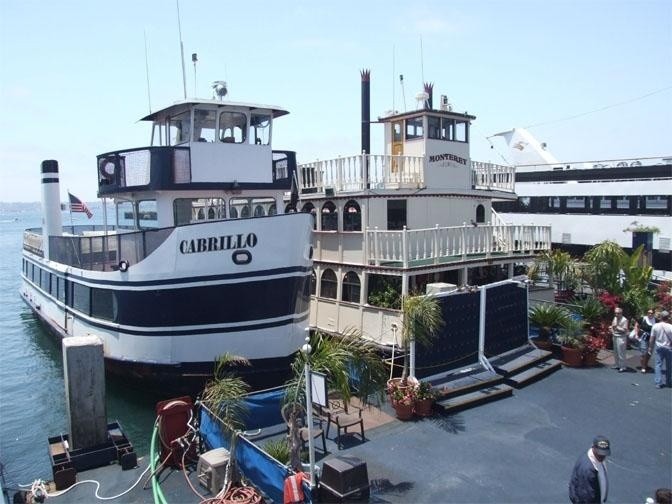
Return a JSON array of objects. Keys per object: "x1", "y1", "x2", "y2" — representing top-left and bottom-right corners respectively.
[
  {"x1": 386, "y1": 285, "x2": 447, "y2": 409},
  {"x1": 526, "y1": 247, "x2": 608, "y2": 367}
]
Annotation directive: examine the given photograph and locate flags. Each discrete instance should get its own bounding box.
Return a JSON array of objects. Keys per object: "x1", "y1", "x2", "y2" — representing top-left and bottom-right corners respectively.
[{"x1": 69, "y1": 192, "x2": 94, "y2": 219}]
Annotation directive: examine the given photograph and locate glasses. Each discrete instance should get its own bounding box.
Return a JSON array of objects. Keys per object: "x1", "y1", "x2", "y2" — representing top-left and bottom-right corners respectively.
[
  {"x1": 614, "y1": 312, "x2": 622, "y2": 315},
  {"x1": 647, "y1": 312, "x2": 654, "y2": 315}
]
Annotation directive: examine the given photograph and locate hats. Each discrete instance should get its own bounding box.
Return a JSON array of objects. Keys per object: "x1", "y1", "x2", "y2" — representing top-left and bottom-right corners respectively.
[{"x1": 591, "y1": 435, "x2": 611, "y2": 457}]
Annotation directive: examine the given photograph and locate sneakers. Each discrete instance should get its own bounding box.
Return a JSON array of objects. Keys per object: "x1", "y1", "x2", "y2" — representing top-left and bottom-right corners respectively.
[
  {"x1": 611, "y1": 364, "x2": 627, "y2": 373},
  {"x1": 641, "y1": 366, "x2": 647, "y2": 373}
]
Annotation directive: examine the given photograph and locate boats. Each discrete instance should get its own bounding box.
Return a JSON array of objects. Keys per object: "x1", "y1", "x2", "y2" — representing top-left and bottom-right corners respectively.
[{"x1": 21, "y1": 79, "x2": 317, "y2": 396}]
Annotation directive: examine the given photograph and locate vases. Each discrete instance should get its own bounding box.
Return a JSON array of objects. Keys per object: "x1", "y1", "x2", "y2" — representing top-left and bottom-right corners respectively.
[{"x1": 395, "y1": 397, "x2": 436, "y2": 421}]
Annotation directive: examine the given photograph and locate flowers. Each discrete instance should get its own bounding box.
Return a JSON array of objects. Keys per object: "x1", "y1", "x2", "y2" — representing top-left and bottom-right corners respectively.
[{"x1": 384, "y1": 380, "x2": 443, "y2": 408}]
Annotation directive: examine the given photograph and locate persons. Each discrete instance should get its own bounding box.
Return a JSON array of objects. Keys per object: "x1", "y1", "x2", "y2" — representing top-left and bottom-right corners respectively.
[
  {"x1": 653, "y1": 486, "x2": 672, "y2": 504},
  {"x1": 633, "y1": 308, "x2": 659, "y2": 374},
  {"x1": 607, "y1": 307, "x2": 629, "y2": 372},
  {"x1": 568, "y1": 436, "x2": 612, "y2": 503},
  {"x1": 647, "y1": 310, "x2": 672, "y2": 389}
]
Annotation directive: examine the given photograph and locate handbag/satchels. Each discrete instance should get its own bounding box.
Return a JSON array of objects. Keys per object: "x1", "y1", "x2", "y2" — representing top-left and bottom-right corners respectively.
[{"x1": 627, "y1": 328, "x2": 639, "y2": 343}]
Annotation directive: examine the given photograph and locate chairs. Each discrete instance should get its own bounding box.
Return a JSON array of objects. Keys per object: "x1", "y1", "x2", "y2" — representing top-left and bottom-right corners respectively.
[{"x1": 281, "y1": 390, "x2": 365, "y2": 459}]
